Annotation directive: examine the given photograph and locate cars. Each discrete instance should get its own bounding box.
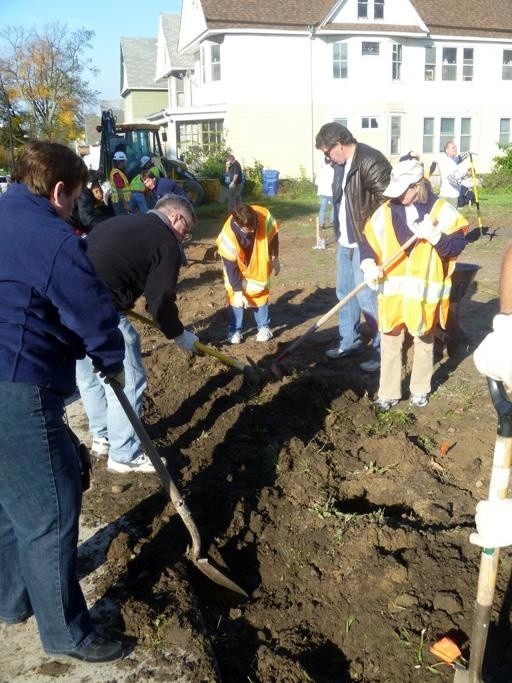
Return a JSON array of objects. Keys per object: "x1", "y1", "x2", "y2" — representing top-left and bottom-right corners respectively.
[{"x1": 0, "y1": 175, "x2": 12, "y2": 195}]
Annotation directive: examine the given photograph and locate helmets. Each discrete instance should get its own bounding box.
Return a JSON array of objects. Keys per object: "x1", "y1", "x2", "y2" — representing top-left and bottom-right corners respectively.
[{"x1": 113, "y1": 151, "x2": 127, "y2": 161}]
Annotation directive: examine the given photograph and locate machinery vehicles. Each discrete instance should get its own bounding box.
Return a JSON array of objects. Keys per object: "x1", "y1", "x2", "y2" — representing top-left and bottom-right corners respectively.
[{"x1": 86, "y1": 107, "x2": 228, "y2": 207}]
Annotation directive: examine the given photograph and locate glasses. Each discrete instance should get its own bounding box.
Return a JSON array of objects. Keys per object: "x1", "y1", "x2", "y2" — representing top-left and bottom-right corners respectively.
[
  {"x1": 172, "y1": 214, "x2": 193, "y2": 241},
  {"x1": 324, "y1": 143, "x2": 337, "y2": 158}
]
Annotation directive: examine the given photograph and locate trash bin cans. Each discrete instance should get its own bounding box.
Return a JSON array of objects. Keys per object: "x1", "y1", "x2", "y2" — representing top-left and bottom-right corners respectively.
[{"x1": 262, "y1": 170, "x2": 279, "y2": 196}]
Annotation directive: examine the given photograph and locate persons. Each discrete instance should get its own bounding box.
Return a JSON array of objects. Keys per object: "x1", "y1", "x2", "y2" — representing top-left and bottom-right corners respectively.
[
  {"x1": 216, "y1": 202, "x2": 280, "y2": 344},
  {"x1": 359, "y1": 158, "x2": 469, "y2": 411},
  {"x1": 468, "y1": 243, "x2": 511, "y2": 547},
  {"x1": 436, "y1": 143, "x2": 471, "y2": 209},
  {"x1": 1, "y1": 143, "x2": 126, "y2": 665},
  {"x1": 66, "y1": 194, "x2": 200, "y2": 472},
  {"x1": 315, "y1": 158, "x2": 334, "y2": 229},
  {"x1": 226, "y1": 155, "x2": 242, "y2": 217},
  {"x1": 70, "y1": 150, "x2": 193, "y2": 267},
  {"x1": 316, "y1": 123, "x2": 393, "y2": 373}
]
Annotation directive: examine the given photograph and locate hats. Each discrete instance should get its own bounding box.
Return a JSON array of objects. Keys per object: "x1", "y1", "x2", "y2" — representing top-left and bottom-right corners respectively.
[
  {"x1": 382, "y1": 160, "x2": 424, "y2": 199},
  {"x1": 97, "y1": 178, "x2": 113, "y2": 207},
  {"x1": 139, "y1": 156, "x2": 150, "y2": 168}
]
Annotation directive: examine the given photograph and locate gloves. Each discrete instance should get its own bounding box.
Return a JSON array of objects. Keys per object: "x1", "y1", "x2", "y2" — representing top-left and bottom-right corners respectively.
[
  {"x1": 232, "y1": 291, "x2": 250, "y2": 309},
  {"x1": 412, "y1": 213, "x2": 440, "y2": 246},
  {"x1": 272, "y1": 259, "x2": 280, "y2": 276},
  {"x1": 469, "y1": 497, "x2": 512, "y2": 548},
  {"x1": 473, "y1": 313, "x2": 512, "y2": 392},
  {"x1": 359, "y1": 258, "x2": 383, "y2": 292},
  {"x1": 93, "y1": 367, "x2": 125, "y2": 390},
  {"x1": 174, "y1": 331, "x2": 200, "y2": 356}
]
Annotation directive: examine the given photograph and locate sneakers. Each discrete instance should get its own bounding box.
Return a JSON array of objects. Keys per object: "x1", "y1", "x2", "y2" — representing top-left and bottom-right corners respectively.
[
  {"x1": 88, "y1": 434, "x2": 167, "y2": 476},
  {"x1": 62, "y1": 634, "x2": 124, "y2": 664},
  {"x1": 227, "y1": 331, "x2": 244, "y2": 344},
  {"x1": 409, "y1": 392, "x2": 428, "y2": 407},
  {"x1": 360, "y1": 359, "x2": 381, "y2": 372},
  {"x1": 325, "y1": 347, "x2": 352, "y2": 358},
  {"x1": 256, "y1": 327, "x2": 274, "y2": 341},
  {"x1": 373, "y1": 398, "x2": 400, "y2": 412}
]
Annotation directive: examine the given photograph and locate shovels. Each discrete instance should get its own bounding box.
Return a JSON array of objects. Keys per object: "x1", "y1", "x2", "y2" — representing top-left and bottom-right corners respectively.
[
  {"x1": 85, "y1": 351, "x2": 251, "y2": 597},
  {"x1": 453, "y1": 377, "x2": 512, "y2": 683}
]
